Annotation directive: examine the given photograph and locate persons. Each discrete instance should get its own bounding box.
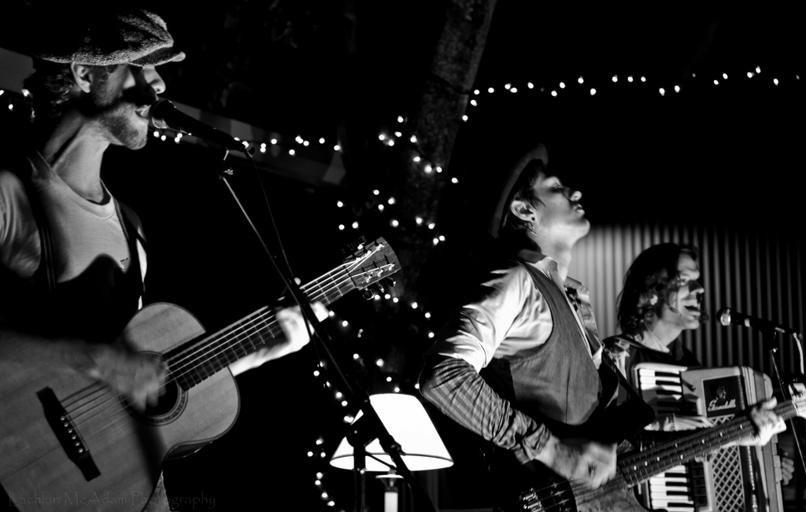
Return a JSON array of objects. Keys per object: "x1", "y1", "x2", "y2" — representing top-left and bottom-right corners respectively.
[
  {"x1": 599, "y1": 241, "x2": 799, "y2": 490},
  {"x1": 416, "y1": 140, "x2": 788, "y2": 512},
  {"x1": 0, "y1": 9, "x2": 334, "y2": 511}
]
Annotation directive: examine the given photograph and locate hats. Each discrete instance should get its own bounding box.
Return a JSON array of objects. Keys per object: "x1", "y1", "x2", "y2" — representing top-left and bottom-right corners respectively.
[
  {"x1": 36, "y1": 10, "x2": 186, "y2": 67},
  {"x1": 491, "y1": 144, "x2": 548, "y2": 238}
]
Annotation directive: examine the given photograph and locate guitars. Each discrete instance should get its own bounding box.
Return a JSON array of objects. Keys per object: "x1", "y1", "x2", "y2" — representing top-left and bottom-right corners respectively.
[
  {"x1": 1, "y1": 237, "x2": 402, "y2": 512},
  {"x1": 460, "y1": 383, "x2": 806, "y2": 512}
]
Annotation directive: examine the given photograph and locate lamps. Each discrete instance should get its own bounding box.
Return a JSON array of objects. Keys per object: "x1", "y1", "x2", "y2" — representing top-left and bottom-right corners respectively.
[{"x1": 329, "y1": 391, "x2": 455, "y2": 512}]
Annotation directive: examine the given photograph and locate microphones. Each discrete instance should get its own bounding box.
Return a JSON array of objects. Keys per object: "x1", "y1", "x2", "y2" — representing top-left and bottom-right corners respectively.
[
  {"x1": 715, "y1": 306, "x2": 788, "y2": 335},
  {"x1": 149, "y1": 99, "x2": 244, "y2": 154}
]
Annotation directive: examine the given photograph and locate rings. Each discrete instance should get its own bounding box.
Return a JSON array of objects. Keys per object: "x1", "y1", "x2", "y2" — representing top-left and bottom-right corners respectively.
[{"x1": 587, "y1": 463, "x2": 597, "y2": 477}]
